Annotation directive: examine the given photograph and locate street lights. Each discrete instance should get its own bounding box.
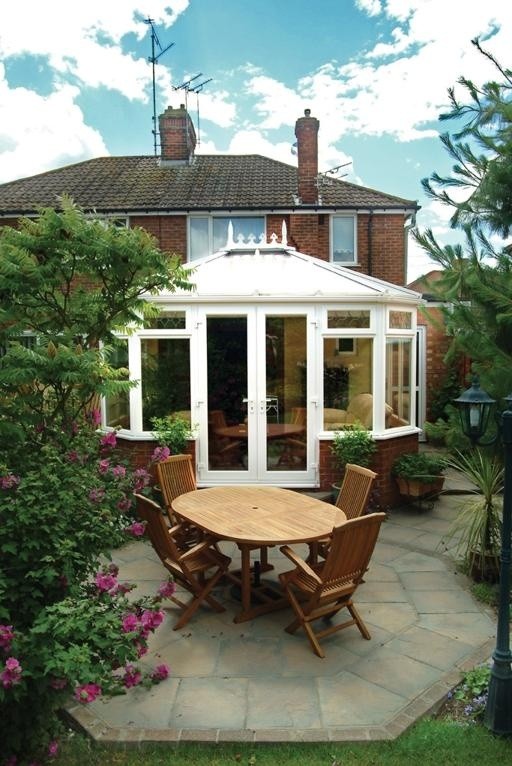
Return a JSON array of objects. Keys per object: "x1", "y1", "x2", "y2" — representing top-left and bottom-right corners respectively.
[{"x1": 453, "y1": 368, "x2": 512, "y2": 742}]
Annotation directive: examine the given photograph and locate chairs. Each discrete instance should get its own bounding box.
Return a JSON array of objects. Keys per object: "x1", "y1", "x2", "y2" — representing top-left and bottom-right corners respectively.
[
  {"x1": 206, "y1": 406, "x2": 307, "y2": 470},
  {"x1": 318, "y1": 464, "x2": 378, "y2": 547},
  {"x1": 156, "y1": 454, "x2": 230, "y2": 574},
  {"x1": 278, "y1": 512, "x2": 386, "y2": 658},
  {"x1": 134, "y1": 494, "x2": 232, "y2": 631}
]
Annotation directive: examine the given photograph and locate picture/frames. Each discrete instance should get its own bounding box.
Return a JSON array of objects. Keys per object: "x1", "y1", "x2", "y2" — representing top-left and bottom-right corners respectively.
[{"x1": 336, "y1": 324, "x2": 358, "y2": 356}]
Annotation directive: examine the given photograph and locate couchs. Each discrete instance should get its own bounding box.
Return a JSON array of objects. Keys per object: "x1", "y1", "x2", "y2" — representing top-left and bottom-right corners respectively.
[{"x1": 325, "y1": 392, "x2": 393, "y2": 431}]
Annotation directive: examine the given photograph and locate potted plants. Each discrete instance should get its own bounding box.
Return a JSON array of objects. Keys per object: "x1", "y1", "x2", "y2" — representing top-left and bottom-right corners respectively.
[
  {"x1": 394, "y1": 444, "x2": 453, "y2": 500},
  {"x1": 327, "y1": 419, "x2": 503, "y2": 583}
]
